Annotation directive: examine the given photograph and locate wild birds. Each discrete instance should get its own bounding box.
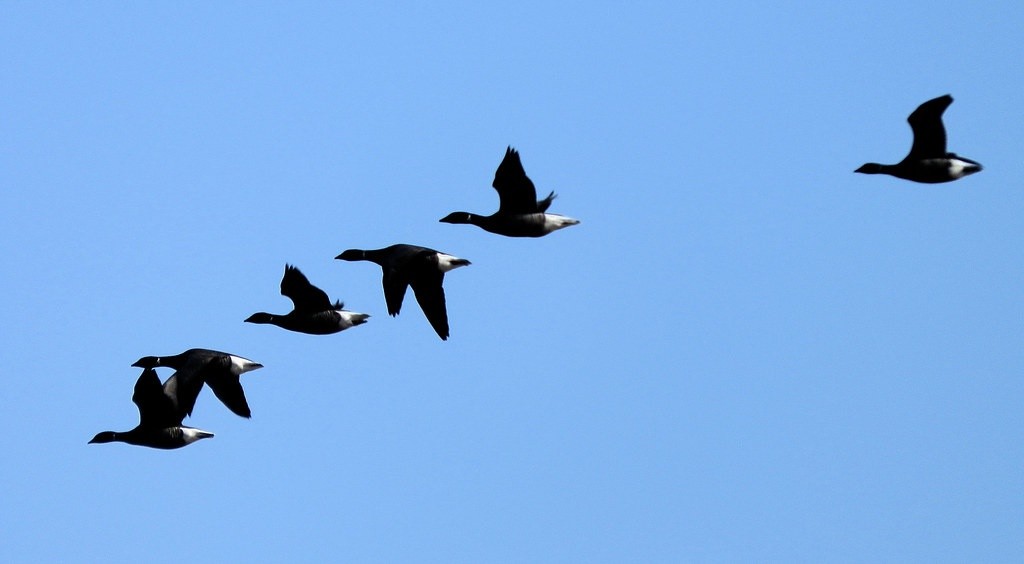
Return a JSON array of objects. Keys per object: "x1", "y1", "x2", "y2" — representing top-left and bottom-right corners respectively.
[
  {"x1": 854, "y1": 94, "x2": 983, "y2": 184},
  {"x1": 334, "y1": 244, "x2": 473, "y2": 341},
  {"x1": 439, "y1": 145, "x2": 580, "y2": 237},
  {"x1": 88, "y1": 367, "x2": 214, "y2": 450},
  {"x1": 131, "y1": 349, "x2": 263, "y2": 419},
  {"x1": 244, "y1": 263, "x2": 372, "y2": 335}
]
